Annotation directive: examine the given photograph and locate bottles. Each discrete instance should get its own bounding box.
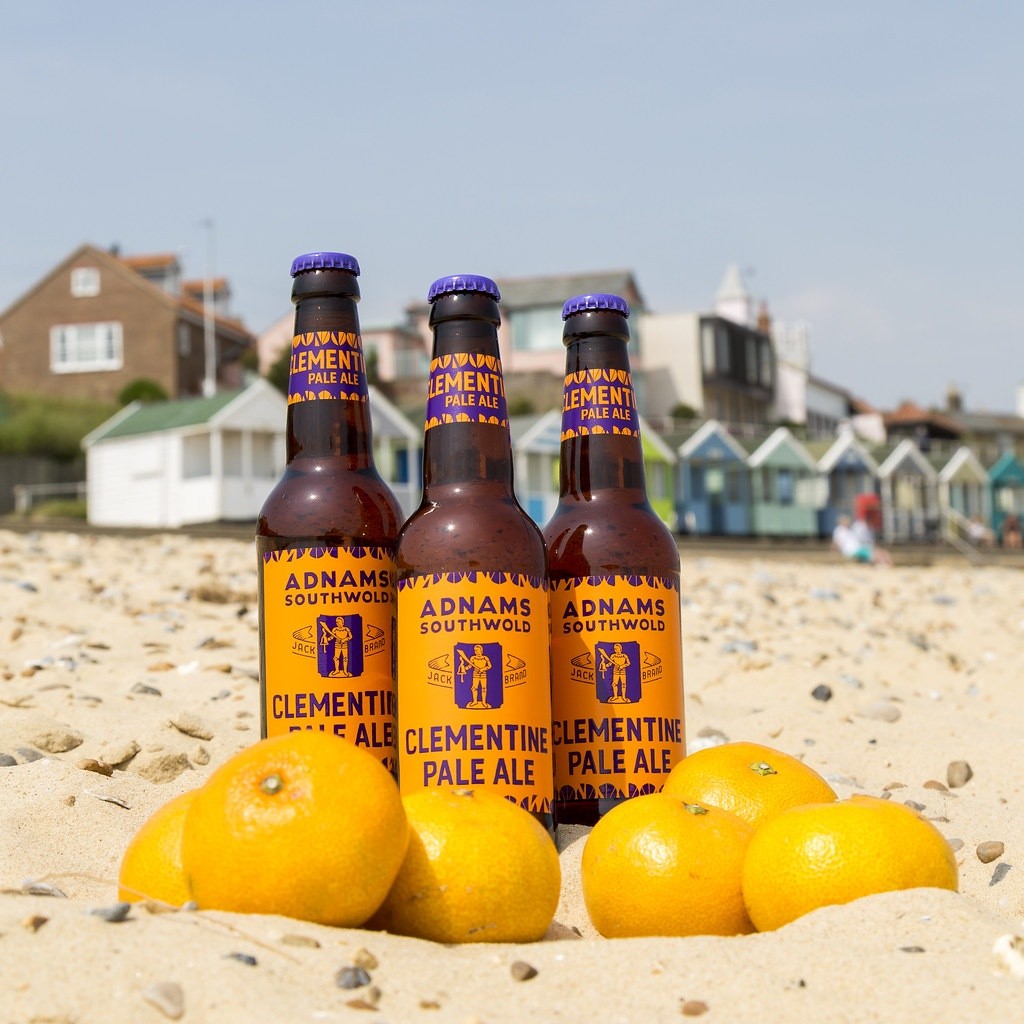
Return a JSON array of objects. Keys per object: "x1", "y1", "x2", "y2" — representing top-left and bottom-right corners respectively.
[
  {"x1": 258, "y1": 253, "x2": 403, "y2": 790},
  {"x1": 398, "y1": 276, "x2": 554, "y2": 846},
  {"x1": 541, "y1": 295, "x2": 686, "y2": 828}
]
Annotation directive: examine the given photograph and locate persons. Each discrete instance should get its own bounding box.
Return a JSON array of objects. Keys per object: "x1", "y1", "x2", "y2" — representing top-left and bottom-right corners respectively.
[
  {"x1": 831, "y1": 508, "x2": 892, "y2": 568},
  {"x1": 965, "y1": 512, "x2": 1023, "y2": 550}
]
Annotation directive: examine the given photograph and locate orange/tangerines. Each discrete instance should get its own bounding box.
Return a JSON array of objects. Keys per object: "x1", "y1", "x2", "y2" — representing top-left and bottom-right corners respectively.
[
  {"x1": 741, "y1": 795, "x2": 958, "y2": 932},
  {"x1": 581, "y1": 791, "x2": 752, "y2": 939},
  {"x1": 663, "y1": 741, "x2": 838, "y2": 828},
  {"x1": 117, "y1": 729, "x2": 562, "y2": 944}
]
